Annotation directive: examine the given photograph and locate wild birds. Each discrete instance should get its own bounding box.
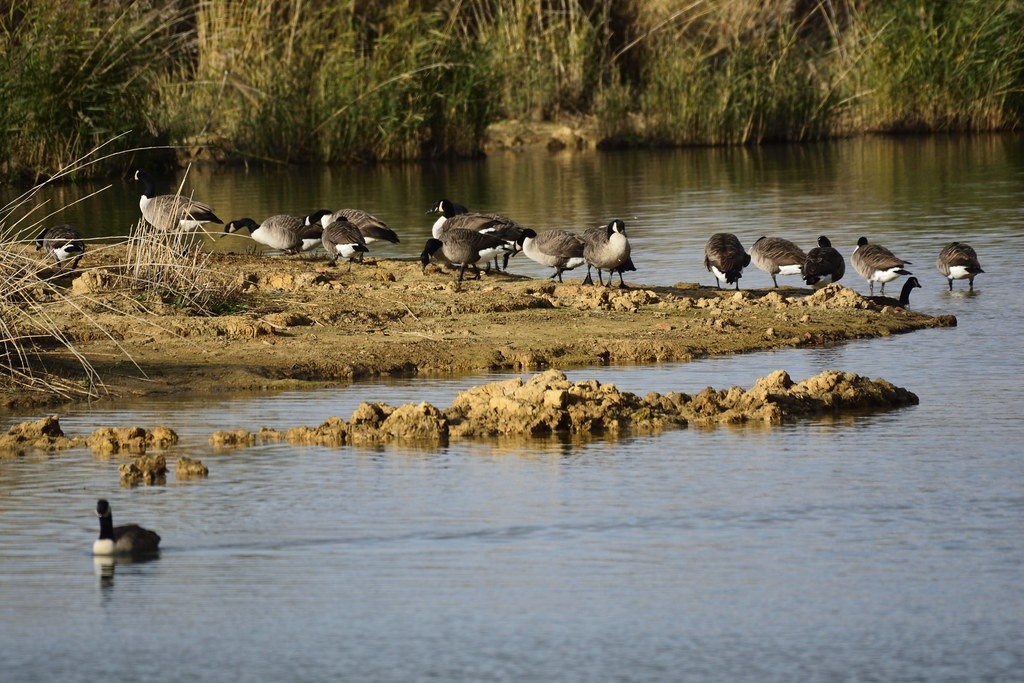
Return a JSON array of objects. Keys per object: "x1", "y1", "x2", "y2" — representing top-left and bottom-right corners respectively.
[
  {"x1": 849, "y1": 236, "x2": 914, "y2": 293},
  {"x1": 748, "y1": 236, "x2": 808, "y2": 289},
  {"x1": 422, "y1": 199, "x2": 638, "y2": 289},
  {"x1": 799, "y1": 235, "x2": 846, "y2": 294},
  {"x1": 862, "y1": 277, "x2": 922, "y2": 311},
  {"x1": 303, "y1": 208, "x2": 401, "y2": 272},
  {"x1": 36, "y1": 224, "x2": 85, "y2": 278},
  {"x1": 92, "y1": 498, "x2": 161, "y2": 557},
  {"x1": 129, "y1": 168, "x2": 225, "y2": 256},
  {"x1": 220, "y1": 214, "x2": 324, "y2": 256},
  {"x1": 936, "y1": 241, "x2": 986, "y2": 291},
  {"x1": 703, "y1": 232, "x2": 752, "y2": 290}
]
